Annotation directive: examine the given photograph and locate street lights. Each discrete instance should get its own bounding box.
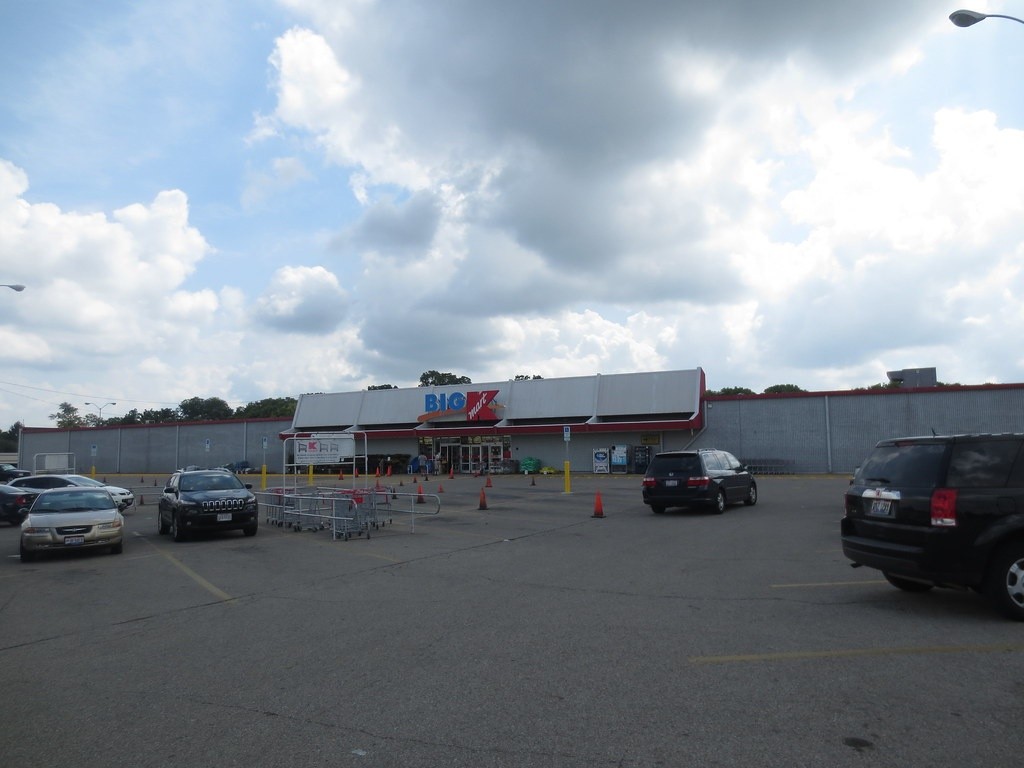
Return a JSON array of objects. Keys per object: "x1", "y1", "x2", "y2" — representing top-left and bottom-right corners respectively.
[{"x1": 85, "y1": 402, "x2": 116, "y2": 424}]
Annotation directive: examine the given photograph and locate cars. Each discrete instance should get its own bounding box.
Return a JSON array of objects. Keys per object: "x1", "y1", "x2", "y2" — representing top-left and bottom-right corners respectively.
[
  {"x1": 7, "y1": 471, "x2": 135, "y2": 511},
  {"x1": 0, "y1": 482, "x2": 40, "y2": 525},
  {"x1": 18, "y1": 485, "x2": 127, "y2": 563},
  {"x1": 0, "y1": 463, "x2": 32, "y2": 482}
]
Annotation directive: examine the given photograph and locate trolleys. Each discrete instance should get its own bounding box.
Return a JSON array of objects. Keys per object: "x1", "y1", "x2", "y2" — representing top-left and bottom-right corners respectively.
[{"x1": 264, "y1": 484, "x2": 394, "y2": 541}]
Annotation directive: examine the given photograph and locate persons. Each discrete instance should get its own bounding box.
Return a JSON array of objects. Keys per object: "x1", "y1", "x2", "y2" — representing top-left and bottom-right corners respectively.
[
  {"x1": 418, "y1": 452, "x2": 428, "y2": 476},
  {"x1": 434, "y1": 452, "x2": 439, "y2": 473}
]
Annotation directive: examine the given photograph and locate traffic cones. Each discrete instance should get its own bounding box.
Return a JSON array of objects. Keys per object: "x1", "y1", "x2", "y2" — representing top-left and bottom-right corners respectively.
[
  {"x1": 391, "y1": 488, "x2": 399, "y2": 500},
  {"x1": 589, "y1": 489, "x2": 608, "y2": 518},
  {"x1": 387, "y1": 466, "x2": 392, "y2": 477},
  {"x1": 530, "y1": 476, "x2": 536, "y2": 486},
  {"x1": 141, "y1": 476, "x2": 144, "y2": 482},
  {"x1": 102, "y1": 476, "x2": 107, "y2": 483},
  {"x1": 399, "y1": 479, "x2": 404, "y2": 486},
  {"x1": 139, "y1": 494, "x2": 145, "y2": 505},
  {"x1": 412, "y1": 476, "x2": 418, "y2": 483},
  {"x1": 153, "y1": 479, "x2": 158, "y2": 487},
  {"x1": 424, "y1": 474, "x2": 429, "y2": 482},
  {"x1": 375, "y1": 479, "x2": 379, "y2": 486},
  {"x1": 338, "y1": 470, "x2": 344, "y2": 480},
  {"x1": 416, "y1": 483, "x2": 427, "y2": 504},
  {"x1": 355, "y1": 468, "x2": 359, "y2": 478},
  {"x1": 375, "y1": 467, "x2": 381, "y2": 477},
  {"x1": 448, "y1": 468, "x2": 454, "y2": 479},
  {"x1": 437, "y1": 483, "x2": 445, "y2": 493},
  {"x1": 477, "y1": 487, "x2": 490, "y2": 511},
  {"x1": 485, "y1": 472, "x2": 493, "y2": 487}
]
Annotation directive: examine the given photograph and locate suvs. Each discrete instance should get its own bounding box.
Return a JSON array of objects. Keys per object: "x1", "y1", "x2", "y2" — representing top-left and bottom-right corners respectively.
[
  {"x1": 156, "y1": 465, "x2": 259, "y2": 542},
  {"x1": 838, "y1": 429, "x2": 1024, "y2": 622},
  {"x1": 641, "y1": 448, "x2": 759, "y2": 515}
]
